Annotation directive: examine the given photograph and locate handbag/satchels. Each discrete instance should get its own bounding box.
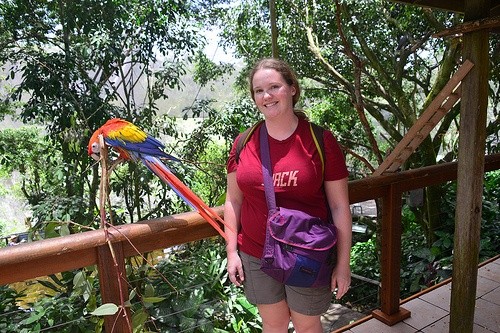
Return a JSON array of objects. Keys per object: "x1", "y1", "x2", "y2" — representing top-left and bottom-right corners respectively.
[{"x1": 260, "y1": 207, "x2": 337, "y2": 287}]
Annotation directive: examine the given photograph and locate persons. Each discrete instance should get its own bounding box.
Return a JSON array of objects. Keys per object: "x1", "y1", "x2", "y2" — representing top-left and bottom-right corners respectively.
[
  {"x1": 8, "y1": 236, "x2": 21, "y2": 247},
  {"x1": 224, "y1": 58, "x2": 352, "y2": 333}
]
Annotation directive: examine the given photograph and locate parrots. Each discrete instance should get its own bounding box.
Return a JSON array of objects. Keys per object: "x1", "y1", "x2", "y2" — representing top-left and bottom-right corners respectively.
[{"x1": 87, "y1": 118, "x2": 236, "y2": 242}]
